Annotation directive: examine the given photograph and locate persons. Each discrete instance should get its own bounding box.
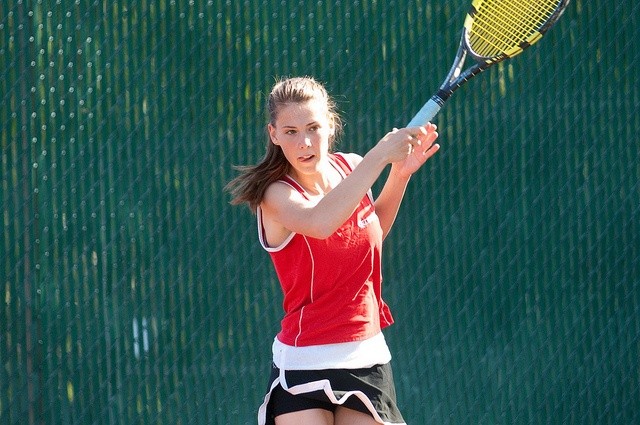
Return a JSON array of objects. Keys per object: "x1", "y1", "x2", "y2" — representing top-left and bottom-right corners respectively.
[{"x1": 218, "y1": 76, "x2": 441, "y2": 425}]
